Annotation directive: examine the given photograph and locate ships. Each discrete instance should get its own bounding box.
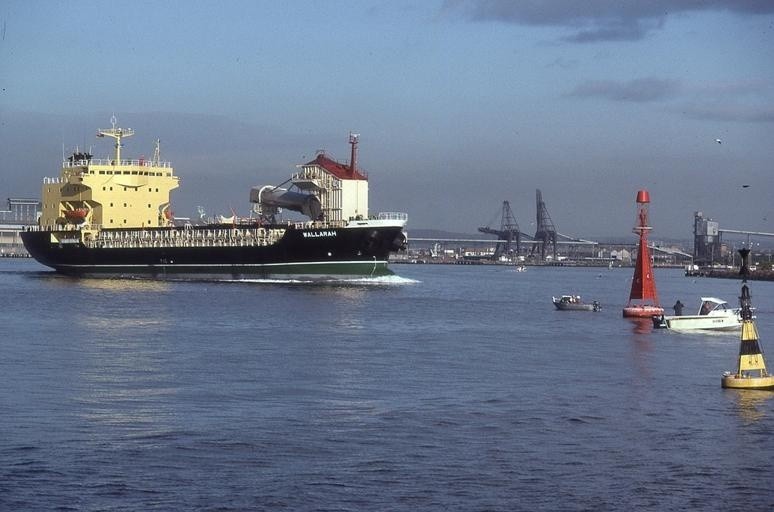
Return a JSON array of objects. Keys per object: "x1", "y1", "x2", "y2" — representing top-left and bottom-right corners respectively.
[{"x1": 20, "y1": 119, "x2": 409, "y2": 285}]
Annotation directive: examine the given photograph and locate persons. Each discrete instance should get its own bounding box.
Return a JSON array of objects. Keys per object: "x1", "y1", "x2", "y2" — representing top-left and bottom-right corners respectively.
[
  {"x1": 699, "y1": 302, "x2": 711, "y2": 315},
  {"x1": 568, "y1": 294, "x2": 577, "y2": 304},
  {"x1": 672, "y1": 300, "x2": 684, "y2": 316}
]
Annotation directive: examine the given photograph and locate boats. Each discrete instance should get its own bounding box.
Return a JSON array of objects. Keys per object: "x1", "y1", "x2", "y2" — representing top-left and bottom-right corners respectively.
[
  {"x1": 551, "y1": 295, "x2": 603, "y2": 313},
  {"x1": 664, "y1": 297, "x2": 757, "y2": 334}
]
